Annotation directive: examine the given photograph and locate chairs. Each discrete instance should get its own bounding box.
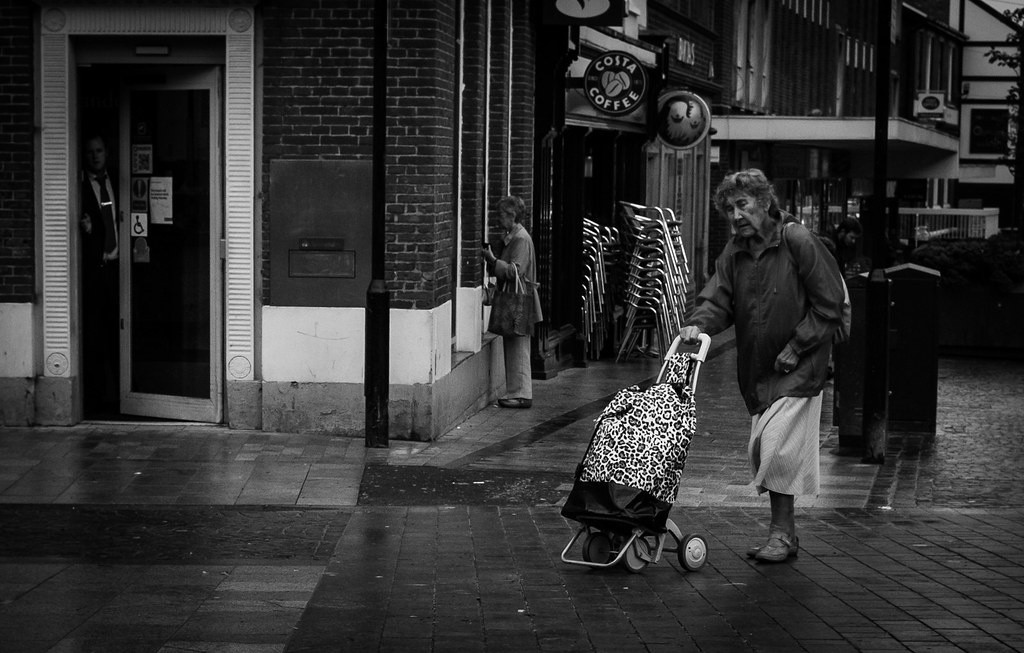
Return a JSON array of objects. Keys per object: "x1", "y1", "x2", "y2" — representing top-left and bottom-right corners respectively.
[{"x1": 580, "y1": 201, "x2": 690, "y2": 369}]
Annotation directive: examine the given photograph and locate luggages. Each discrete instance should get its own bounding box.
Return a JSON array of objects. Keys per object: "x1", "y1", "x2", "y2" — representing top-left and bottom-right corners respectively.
[{"x1": 560, "y1": 333, "x2": 712, "y2": 573}]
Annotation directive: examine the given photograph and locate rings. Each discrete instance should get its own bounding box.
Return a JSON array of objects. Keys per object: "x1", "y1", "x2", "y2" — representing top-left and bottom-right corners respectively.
[{"x1": 785, "y1": 369, "x2": 789, "y2": 373}]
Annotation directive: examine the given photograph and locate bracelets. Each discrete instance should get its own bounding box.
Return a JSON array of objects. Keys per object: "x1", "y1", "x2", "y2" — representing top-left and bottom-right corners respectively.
[{"x1": 492, "y1": 258, "x2": 497, "y2": 264}]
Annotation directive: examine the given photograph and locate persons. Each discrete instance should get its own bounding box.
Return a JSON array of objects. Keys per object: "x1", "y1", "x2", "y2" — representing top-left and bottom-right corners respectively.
[
  {"x1": 76, "y1": 130, "x2": 121, "y2": 413},
  {"x1": 679, "y1": 167, "x2": 844, "y2": 562},
  {"x1": 483, "y1": 195, "x2": 544, "y2": 408},
  {"x1": 818, "y1": 216, "x2": 862, "y2": 381}
]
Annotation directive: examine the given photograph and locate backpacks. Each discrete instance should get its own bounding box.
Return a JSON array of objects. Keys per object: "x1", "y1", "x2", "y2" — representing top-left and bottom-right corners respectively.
[{"x1": 783, "y1": 217, "x2": 851, "y2": 344}]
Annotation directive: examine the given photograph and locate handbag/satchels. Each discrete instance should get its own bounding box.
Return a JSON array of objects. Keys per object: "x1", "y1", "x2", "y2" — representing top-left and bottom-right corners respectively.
[{"x1": 487, "y1": 263, "x2": 529, "y2": 337}]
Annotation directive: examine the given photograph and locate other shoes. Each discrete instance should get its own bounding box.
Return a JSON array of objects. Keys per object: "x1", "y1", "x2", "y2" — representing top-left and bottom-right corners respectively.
[
  {"x1": 497, "y1": 397, "x2": 532, "y2": 410},
  {"x1": 747, "y1": 526, "x2": 801, "y2": 561}
]
